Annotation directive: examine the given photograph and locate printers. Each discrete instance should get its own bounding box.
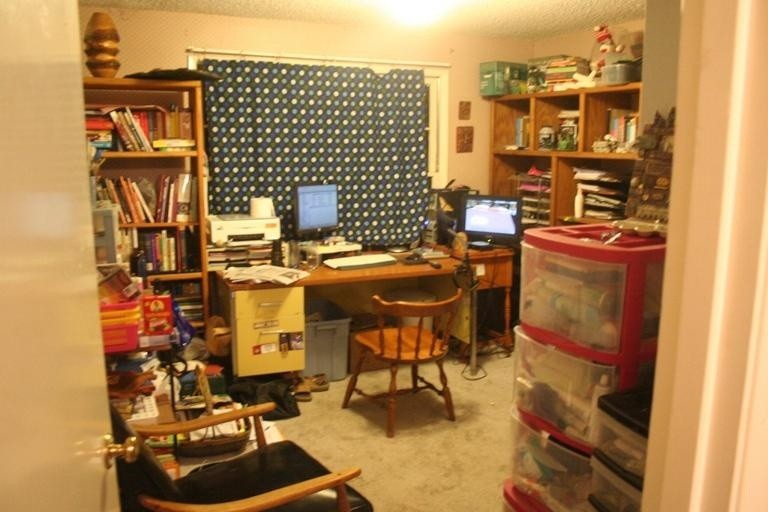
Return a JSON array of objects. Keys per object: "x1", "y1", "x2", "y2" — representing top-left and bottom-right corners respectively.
[{"x1": 208, "y1": 214, "x2": 281, "y2": 246}]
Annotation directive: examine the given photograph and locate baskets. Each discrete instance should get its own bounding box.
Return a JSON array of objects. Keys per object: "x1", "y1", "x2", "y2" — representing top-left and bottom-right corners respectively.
[
  {"x1": 100, "y1": 301, "x2": 141, "y2": 353},
  {"x1": 179, "y1": 365, "x2": 251, "y2": 458}
]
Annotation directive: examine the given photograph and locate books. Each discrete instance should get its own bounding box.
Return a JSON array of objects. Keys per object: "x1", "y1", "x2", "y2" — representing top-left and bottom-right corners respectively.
[
  {"x1": 222, "y1": 264, "x2": 310, "y2": 287},
  {"x1": 90, "y1": 174, "x2": 198, "y2": 223},
  {"x1": 142, "y1": 282, "x2": 202, "y2": 336},
  {"x1": 86, "y1": 106, "x2": 194, "y2": 152},
  {"x1": 505, "y1": 53, "x2": 639, "y2": 226},
  {"x1": 118, "y1": 226, "x2": 197, "y2": 273}
]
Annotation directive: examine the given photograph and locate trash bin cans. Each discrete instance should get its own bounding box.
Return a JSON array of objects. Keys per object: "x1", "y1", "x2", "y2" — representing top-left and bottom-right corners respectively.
[{"x1": 381, "y1": 287, "x2": 438, "y2": 334}]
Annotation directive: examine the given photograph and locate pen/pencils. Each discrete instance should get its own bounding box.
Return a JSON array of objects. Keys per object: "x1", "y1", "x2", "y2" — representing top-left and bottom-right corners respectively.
[{"x1": 604, "y1": 232, "x2": 621, "y2": 245}]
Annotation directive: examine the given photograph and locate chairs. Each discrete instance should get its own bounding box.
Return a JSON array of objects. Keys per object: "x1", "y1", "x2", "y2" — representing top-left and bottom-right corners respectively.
[
  {"x1": 341, "y1": 287, "x2": 465, "y2": 438},
  {"x1": 107, "y1": 399, "x2": 374, "y2": 511}
]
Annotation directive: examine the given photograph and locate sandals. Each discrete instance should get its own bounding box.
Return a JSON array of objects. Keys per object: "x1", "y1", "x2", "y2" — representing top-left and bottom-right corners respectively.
[{"x1": 291, "y1": 373, "x2": 329, "y2": 401}]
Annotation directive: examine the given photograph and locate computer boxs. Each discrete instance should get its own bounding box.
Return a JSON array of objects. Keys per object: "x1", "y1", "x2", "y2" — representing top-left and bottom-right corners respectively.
[
  {"x1": 299, "y1": 240, "x2": 363, "y2": 261},
  {"x1": 422, "y1": 186, "x2": 470, "y2": 245}
]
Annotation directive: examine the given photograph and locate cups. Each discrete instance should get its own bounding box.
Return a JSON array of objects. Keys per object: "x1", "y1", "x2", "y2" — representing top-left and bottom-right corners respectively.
[{"x1": 306, "y1": 245, "x2": 319, "y2": 268}]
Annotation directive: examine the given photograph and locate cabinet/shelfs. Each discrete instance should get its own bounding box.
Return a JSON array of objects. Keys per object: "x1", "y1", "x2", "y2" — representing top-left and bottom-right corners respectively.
[
  {"x1": 484, "y1": 82, "x2": 643, "y2": 250},
  {"x1": 81, "y1": 75, "x2": 210, "y2": 333}
]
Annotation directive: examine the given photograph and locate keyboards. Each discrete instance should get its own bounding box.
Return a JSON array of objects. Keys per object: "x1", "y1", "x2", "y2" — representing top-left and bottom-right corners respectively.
[{"x1": 323, "y1": 253, "x2": 399, "y2": 270}]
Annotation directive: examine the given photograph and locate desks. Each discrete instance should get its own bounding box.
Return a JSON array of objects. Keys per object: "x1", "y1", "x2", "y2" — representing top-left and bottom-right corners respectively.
[
  {"x1": 209, "y1": 245, "x2": 473, "y2": 377},
  {"x1": 422, "y1": 240, "x2": 516, "y2": 365}
]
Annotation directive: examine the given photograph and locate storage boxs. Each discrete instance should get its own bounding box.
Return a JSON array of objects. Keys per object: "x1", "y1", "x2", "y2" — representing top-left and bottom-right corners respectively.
[
  {"x1": 478, "y1": 62, "x2": 527, "y2": 97},
  {"x1": 292, "y1": 296, "x2": 352, "y2": 383}
]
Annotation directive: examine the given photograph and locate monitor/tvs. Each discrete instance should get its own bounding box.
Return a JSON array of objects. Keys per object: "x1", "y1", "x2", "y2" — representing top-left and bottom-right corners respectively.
[
  {"x1": 459, "y1": 194, "x2": 522, "y2": 244},
  {"x1": 293, "y1": 183, "x2": 339, "y2": 239}
]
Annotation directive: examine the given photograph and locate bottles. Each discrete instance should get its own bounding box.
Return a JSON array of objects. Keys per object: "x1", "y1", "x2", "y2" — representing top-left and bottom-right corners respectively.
[
  {"x1": 573, "y1": 183, "x2": 585, "y2": 217},
  {"x1": 536, "y1": 125, "x2": 555, "y2": 151},
  {"x1": 451, "y1": 233, "x2": 468, "y2": 259}
]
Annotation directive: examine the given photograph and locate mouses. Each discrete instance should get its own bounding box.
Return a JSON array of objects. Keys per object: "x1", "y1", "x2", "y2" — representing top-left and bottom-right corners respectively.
[{"x1": 406, "y1": 255, "x2": 420, "y2": 261}]
